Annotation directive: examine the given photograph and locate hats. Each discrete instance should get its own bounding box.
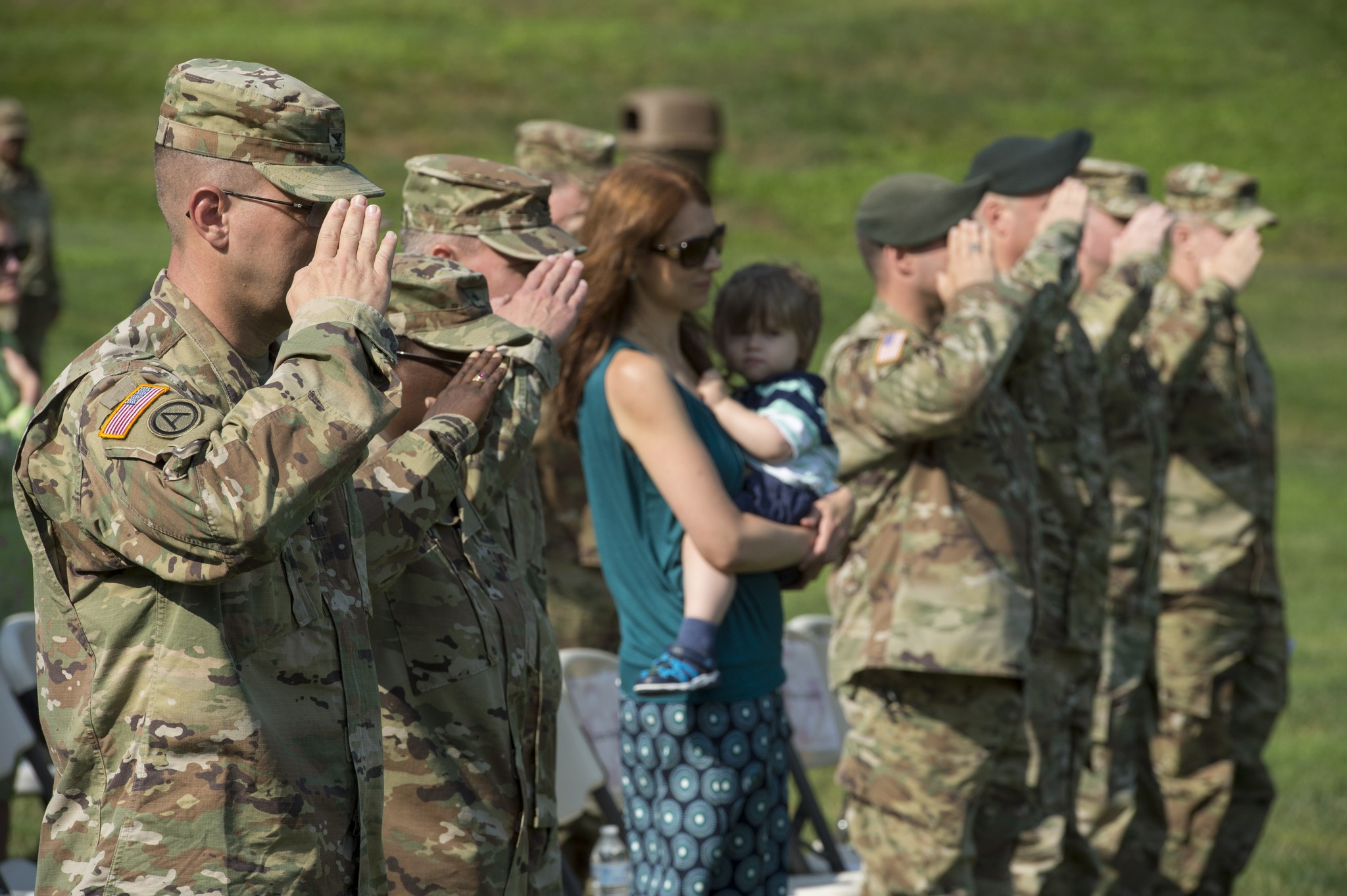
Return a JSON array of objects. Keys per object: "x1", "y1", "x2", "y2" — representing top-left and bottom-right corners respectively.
[
  {"x1": 1075, "y1": 157, "x2": 1152, "y2": 219},
  {"x1": 380, "y1": 253, "x2": 533, "y2": 354},
  {"x1": 154, "y1": 57, "x2": 386, "y2": 203},
  {"x1": 515, "y1": 119, "x2": 615, "y2": 188},
  {"x1": 856, "y1": 175, "x2": 985, "y2": 248},
  {"x1": 0, "y1": 99, "x2": 27, "y2": 142},
  {"x1": 967, "y1": 127, "x2": 1092, "y2": 196},
  {"x1": 402, "y1": 154, "x2": 589, "y2": 262},
  {"x1": 1165, "y1": 161, "x2": 1279, "y2": 234}
]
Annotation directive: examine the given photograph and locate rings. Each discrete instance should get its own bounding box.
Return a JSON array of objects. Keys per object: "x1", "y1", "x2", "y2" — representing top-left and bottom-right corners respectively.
[{"x1": 473, "y1": 373, "x2": 486, "y2": 383}]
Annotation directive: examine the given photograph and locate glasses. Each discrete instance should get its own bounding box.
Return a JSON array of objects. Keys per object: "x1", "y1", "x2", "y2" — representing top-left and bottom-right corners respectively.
[
  {"x1": 642, "y1": 222, "x2": 726, "y2": 269},
  {"x1": 186, "y1": 189, "x2": 333, "y2": 228},
  {"x1": 0, "y1": 241, "x2": 32, "y2": 264},
  {"x1": 395, "y1": 352, "x2": 465, "y2": 371}
]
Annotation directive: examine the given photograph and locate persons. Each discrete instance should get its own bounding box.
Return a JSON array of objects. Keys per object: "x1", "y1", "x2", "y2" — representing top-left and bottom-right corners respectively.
[
  {"x1": 825, "y1": 129, "x2": 1290, "y2": 896},
  {"x1": 0, "y1": 60, "x2": 615, "y2": 896},
  {"x1": 556, "y1": 152, "x2": 856, "y2": 895}
]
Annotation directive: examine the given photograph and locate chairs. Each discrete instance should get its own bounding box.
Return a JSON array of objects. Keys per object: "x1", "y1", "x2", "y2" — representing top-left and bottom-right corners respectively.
[
  {"x1": 0, "y1": 610, "x2": 51, "y2": 896},
  {"x1": 558, "y1": 612, "x2": 864, "y2": 896}
]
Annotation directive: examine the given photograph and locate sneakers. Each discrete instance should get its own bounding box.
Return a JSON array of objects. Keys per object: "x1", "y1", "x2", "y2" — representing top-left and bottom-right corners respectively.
[{"x1": 634, "y1": 644, "x2": 717, "y2": 696}]
